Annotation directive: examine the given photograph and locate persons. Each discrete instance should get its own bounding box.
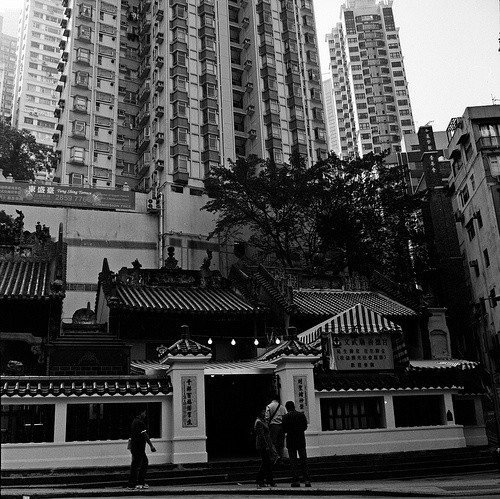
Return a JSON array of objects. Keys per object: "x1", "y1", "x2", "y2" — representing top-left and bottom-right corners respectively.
[
  {"x1": 265, "y1": 394, "x2": 287, "y2": 463},
  {"x1": 254, "y1": 407, "x2": 278, "y2": 487},
  {"x1": 281, "y1": 401, "x2": 311, "y2": 488},
  {"x1": 127, "y1": 406, "x2": 156, "y2": 489}
]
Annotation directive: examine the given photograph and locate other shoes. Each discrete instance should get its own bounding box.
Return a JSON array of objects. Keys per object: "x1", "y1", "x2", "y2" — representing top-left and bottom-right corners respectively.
[
  {"x1": 305, "y1": 481, "x2": 311, "y2": 487},
  {"x1": 291, "y1": 481, "x2": 300, "y2": 487},
  {"x1": 127, "y1": 484, "x2": 142, "y2": 490},
  {"x1": 142, "y1": 483, "x2": 149, "y2": 489}
]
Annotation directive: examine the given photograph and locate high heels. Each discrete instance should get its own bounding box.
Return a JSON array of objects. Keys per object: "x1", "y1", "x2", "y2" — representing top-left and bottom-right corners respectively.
[
  {"x1": 257, "y1": 479, "x2": 266, "y2": 487},
  {"x1": 266, "y1": 479, "x2": 277, "y2": 487}
]
